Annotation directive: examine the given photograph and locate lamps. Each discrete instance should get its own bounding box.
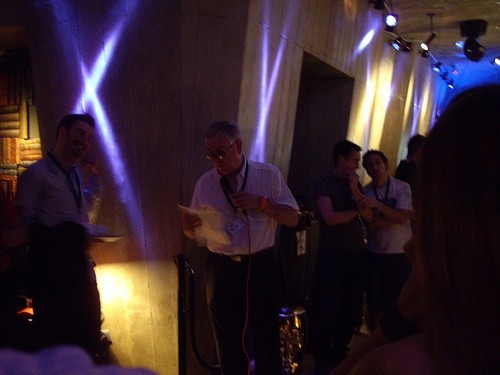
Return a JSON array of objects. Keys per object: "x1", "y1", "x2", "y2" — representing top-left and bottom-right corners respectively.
[{"x1": 383, "y1": 13, "x2": 456, "y2": 89}]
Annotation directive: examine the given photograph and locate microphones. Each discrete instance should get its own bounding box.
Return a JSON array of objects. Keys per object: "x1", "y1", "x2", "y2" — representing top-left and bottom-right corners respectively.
[{"x1": 221, "y1": 177, "x2": 248, "y2": 217}]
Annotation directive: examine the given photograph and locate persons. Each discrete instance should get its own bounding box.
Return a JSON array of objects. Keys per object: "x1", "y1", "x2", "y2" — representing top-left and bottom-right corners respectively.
[
  {"x1": 314, "y1": 141, "x2": 371, "y2": 371},
  {"x1": 325, "y1": 84, "x2": 500, "y2": 375},
  {"x1": 0, "y1": 245, "x2": 156, "y2": 375},
  {"x1": 17, "y1": 113, "x2": 101, "y2": 350},
  {"x1": 359, "y1": 153, "x2": 414, "y2": 332},
  {"x1": 180, "y1": 120, "x2": 300, "y2": 375},
  {"x1": 394, "y1": 135, "x2": 426, "y2": 183}
]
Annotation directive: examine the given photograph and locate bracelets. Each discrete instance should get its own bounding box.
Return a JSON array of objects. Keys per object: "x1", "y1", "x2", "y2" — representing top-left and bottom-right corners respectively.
[
  {"x1": 259, "y1": 195, "x2": 267, "y2": 209},
  {"x1": 352, "y1": 190, "x2": 360, "y2": 194}
]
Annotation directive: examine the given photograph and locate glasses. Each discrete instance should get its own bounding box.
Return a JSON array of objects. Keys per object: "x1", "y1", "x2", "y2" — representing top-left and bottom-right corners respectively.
[{"x1": 205, "y1": 142, "x2": 234, "y2": 161}]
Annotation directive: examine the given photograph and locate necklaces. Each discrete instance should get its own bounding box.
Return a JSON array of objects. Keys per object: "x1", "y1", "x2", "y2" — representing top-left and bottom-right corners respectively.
[
  {"x1": 372, "y1": 176, "x2": 390, "y2": 203},
  {"x1": 46, "y1": 151, "x2": 81, "y2": 213}
]
